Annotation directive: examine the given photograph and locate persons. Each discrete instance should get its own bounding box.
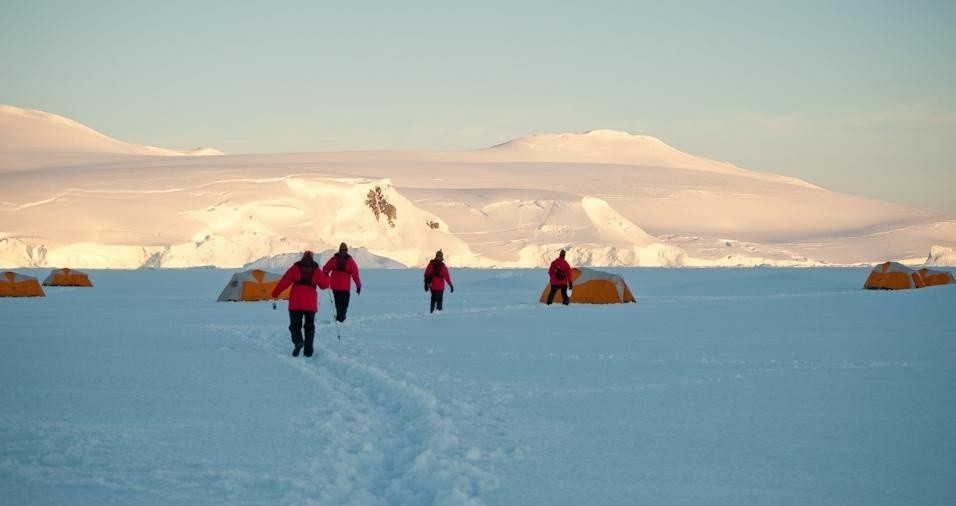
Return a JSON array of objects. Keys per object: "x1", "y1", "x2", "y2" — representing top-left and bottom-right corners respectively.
[
  {"x1": 423, "y1": 249, "x2": 454, "y2": 314},
  {"x1": 546, "y1": 249, "x2": 572, "y2": 306},
  {"x1": 323, "y1": 243, "x2": 361, "y2": 322},
  {"x1": 271, "y1": 251, "x2": 331, "y2": 359}
]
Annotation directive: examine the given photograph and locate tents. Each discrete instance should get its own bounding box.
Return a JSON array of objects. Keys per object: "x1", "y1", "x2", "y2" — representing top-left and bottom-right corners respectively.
[
  {"x1": 42, "y1": 267, "x2": 93, "y2": 286},
  {"x1": 917, "y1": 268, "x2": 956, "y2": 288},
  {"x1": 539, "y1": 266, "x2": 636, "y2": 304},
  {"x1": 864, "y1": 261, "x2": 925, "y2": 290},
  {"x1": 216, "y1": 269, "x2": 297, "y2": 301},
  {"x1": 0, "y1": 272, "x2": 46, "y2": 296}
]
